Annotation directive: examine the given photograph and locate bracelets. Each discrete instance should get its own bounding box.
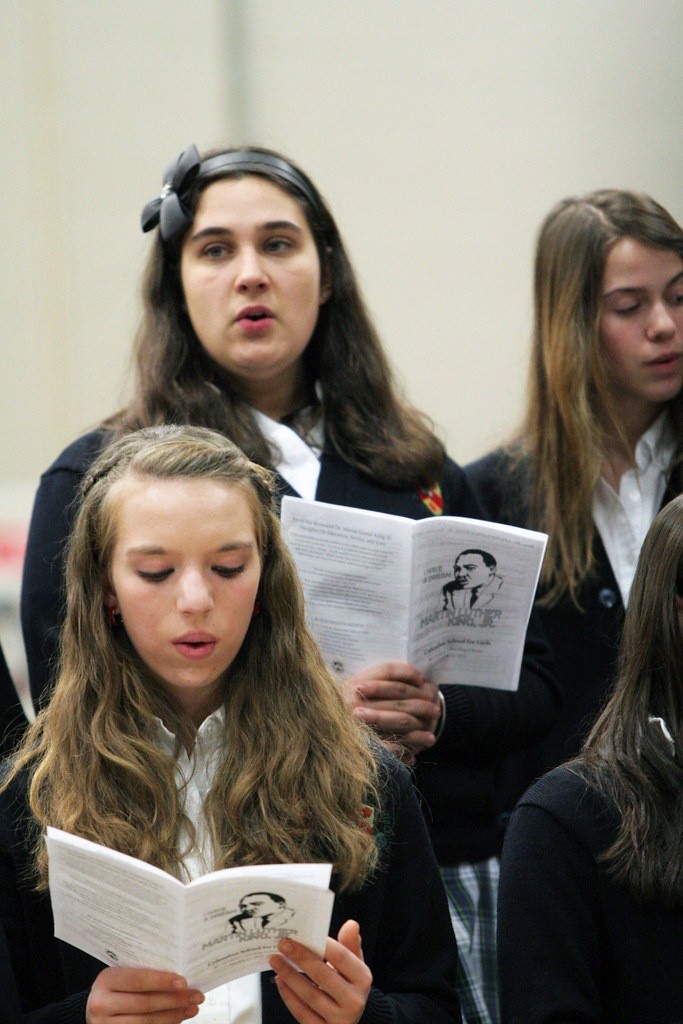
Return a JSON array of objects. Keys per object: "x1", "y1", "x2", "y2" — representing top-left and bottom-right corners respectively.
[{"x1": 432, "y1": 689, "x2": 447, "y2": 751}]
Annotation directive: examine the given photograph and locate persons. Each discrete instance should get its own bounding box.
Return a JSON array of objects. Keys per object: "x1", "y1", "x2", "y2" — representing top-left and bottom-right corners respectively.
[
  {"x1": 498, "y1": 494, "x2": 683, "y2": 1024},
  {"x1": 0, "y1": 424, "x2": 461, "y2": 1024},
  {"x1": 20, "y1": 147, "x2": 683, "y2": 1024}
]
